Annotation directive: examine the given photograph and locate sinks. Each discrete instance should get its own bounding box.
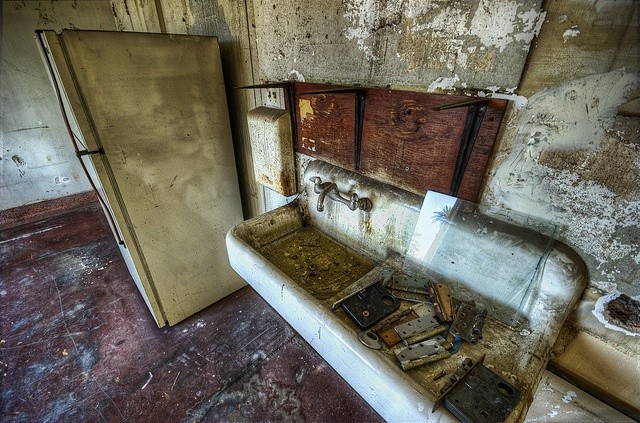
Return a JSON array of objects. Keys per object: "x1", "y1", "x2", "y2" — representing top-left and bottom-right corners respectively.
[
  {"x1": 241, "y1": 207, "x2": 393, "y2": 300},
  {"x1": 330, "y1": 268, "x2": 533, "y2": 421}
]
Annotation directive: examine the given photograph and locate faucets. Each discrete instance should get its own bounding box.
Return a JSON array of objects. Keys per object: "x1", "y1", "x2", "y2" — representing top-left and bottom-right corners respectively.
[{"x1": 317, "y1": 181, "x2": 339, "y2": 211}]
